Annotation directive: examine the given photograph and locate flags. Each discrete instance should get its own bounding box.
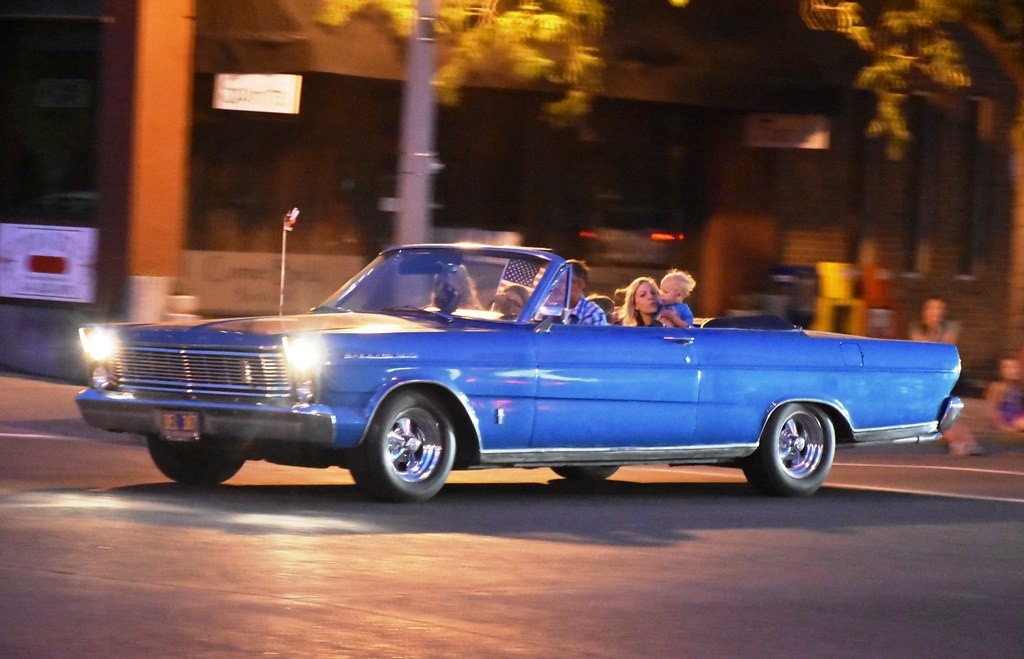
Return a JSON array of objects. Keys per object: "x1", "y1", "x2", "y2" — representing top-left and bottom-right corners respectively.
[{"x1": 498, "y1": 258, "x2": 560, "y2": 306}]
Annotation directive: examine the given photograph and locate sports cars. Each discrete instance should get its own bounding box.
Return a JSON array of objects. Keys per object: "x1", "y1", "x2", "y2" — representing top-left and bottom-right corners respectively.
[{"x1": 74, "y1": 243, "x2": 963, "y2": 497}]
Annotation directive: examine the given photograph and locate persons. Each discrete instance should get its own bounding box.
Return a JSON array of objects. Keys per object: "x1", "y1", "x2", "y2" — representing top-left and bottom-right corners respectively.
[
  {"x1": 987, "y1": 357, "x2": 1024, "y2": 433},
  {"x1": 421, "y1": 264, "x2": 482, "y2": 311},
  {"x1": 536, "y1": 259, "x2": 607, "y2": 326},
  {"x1": 619, "y1": 268, "x2": 697, "y2": 328},
  {"x1": 907, "y1": 298, "x2": 986, "y2": 456}
]
